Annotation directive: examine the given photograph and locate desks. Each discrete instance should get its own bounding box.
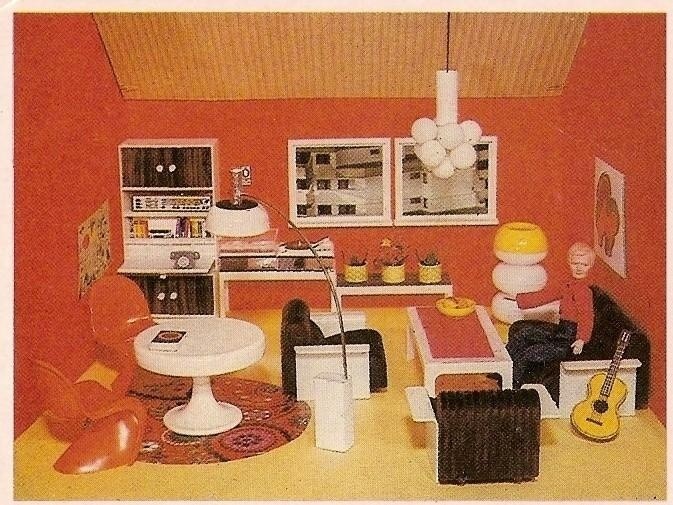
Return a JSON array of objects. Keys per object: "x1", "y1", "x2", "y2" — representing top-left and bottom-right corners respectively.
[
  {"x1": 218, "y1": 270, "x2": 453, "y2": 317},
  {"x1": 133, "y1": 317, "x2": 266, "y2": 438}
]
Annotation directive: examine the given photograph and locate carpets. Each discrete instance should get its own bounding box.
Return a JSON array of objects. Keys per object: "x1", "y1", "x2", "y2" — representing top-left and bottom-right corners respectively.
[{"x1": 125, "y1": 378, "x2": 310, "y2": 466}]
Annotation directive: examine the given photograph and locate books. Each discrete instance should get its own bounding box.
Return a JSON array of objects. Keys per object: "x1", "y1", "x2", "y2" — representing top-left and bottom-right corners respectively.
[{"x1": 132, "y1": 218, "x2": 206, "y2": 238}]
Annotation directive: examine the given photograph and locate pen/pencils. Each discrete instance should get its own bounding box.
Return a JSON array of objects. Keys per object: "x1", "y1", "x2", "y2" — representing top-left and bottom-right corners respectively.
[
  {"x1": 341, "y1": 251, "x2": 369, "y2": 266},
  {"x1": 381, "y1": 255, "x2": 409, "y2": 266}
]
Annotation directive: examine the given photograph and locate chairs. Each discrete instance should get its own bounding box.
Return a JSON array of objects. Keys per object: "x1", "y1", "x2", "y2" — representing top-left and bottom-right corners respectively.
[
  {"x1": 279, "y1": 297, "x2": 387, "y2": 399},
  {"x1": 31, "y1": 274, "x2": 159, "y2": 475}
]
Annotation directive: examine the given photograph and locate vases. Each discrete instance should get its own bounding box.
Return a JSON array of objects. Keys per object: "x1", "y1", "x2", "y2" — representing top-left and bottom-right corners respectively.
[{"x1": 380, "y1": 262, "x2": 404, "y2": 284}]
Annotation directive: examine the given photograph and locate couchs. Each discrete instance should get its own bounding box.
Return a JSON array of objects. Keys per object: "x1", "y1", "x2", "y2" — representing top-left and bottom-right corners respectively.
[{"x1": 521, "y1": 284, "x2": 650, "y2": 418}]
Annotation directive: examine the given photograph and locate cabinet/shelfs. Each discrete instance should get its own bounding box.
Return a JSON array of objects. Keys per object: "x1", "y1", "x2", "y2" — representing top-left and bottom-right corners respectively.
[{"x1": 117, "y1": 137, "x2": 218, "y2": 319}]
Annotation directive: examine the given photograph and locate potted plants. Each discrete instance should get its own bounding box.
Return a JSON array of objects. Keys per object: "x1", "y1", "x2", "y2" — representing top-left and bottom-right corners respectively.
[
  {"x1": 417, "y1": 251, "x2": 441, "y2": 284},
  {"x1": 343, "y1": 254, "x2": 367, "y2": 283}
]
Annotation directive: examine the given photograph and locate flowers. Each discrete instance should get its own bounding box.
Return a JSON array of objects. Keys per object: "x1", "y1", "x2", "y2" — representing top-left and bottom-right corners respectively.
[{"x1": 376, "y1": 238, "x2": 408, "y2": 266}]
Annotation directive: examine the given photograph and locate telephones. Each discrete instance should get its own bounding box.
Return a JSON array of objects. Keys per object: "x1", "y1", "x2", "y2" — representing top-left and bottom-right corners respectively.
[{"x1": 169, "y1": 251, "x2": 200, "y2": 269}]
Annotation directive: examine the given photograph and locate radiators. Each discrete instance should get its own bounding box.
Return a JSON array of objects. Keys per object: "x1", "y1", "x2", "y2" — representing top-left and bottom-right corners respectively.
[{"x1": 405, "y1": 384, "x2": 560, "y2": 486}]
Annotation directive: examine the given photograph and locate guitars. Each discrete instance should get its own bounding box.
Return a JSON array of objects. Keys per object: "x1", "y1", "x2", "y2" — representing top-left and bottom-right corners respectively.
[{"x1": 570, "y1": 328, "x2": 632, "y2": 443}]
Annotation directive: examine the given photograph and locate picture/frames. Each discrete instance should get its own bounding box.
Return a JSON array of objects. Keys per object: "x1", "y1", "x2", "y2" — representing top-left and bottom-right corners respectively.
[{"x1": 286, "y1": 135, "x2": 499, "y2": 229}]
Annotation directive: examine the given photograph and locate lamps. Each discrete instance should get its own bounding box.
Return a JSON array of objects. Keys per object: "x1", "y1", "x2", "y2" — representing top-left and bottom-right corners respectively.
[
  {"x1": 204, "y1": 168, "x2": 353, "y2": 454},
  {"x1": 409, "y1": 12, "x2": 481, "y2": 178}
]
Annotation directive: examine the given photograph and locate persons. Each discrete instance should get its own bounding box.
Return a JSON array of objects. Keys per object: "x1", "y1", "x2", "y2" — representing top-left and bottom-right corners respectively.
[{"x1": 505, "y1": 241, "x2": 596, "y2": 390}]
